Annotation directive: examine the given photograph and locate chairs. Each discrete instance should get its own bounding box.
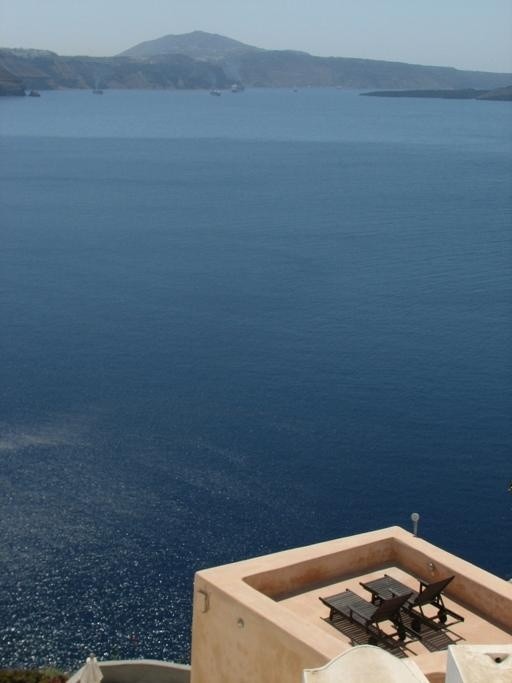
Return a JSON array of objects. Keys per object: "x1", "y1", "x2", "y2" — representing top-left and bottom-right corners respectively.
[
  {"x1": 359, "y1": 573, "x2": 454, "y2": 621},
  {"x1": 318, "y1": 587, "x2": 413, "y2": 640}
]
